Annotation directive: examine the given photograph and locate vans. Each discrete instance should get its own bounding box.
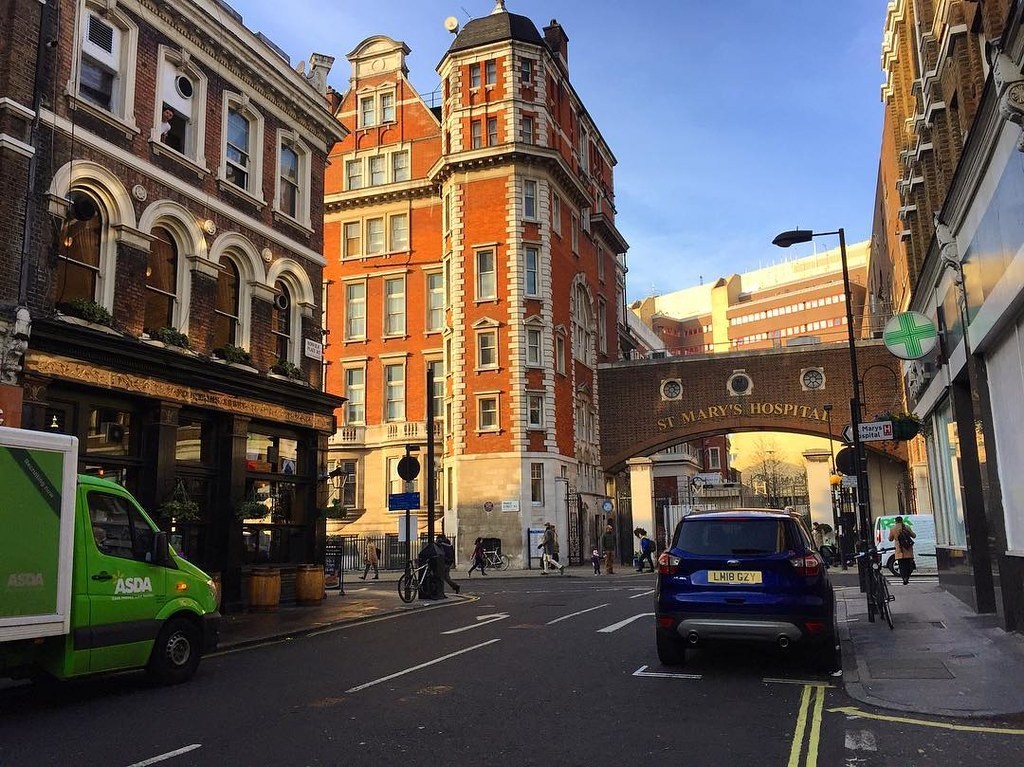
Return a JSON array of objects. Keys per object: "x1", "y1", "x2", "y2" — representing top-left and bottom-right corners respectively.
[
  {"x1": 0, "y1": 425, "x2": 223, "y2": 681},
  {"x1": 873, "y1": 514, "x2": 939, "y2": 578}
]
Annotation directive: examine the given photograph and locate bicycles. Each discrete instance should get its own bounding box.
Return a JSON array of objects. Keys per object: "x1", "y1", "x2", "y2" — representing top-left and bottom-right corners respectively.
[
  {"x1": 471, "y1": 546, "x2": 509, "y2": 571},
  {"x1": 846, "y1": 546, "x2": 895, "y2": 630},
  {"x1": 397, "y1": 559, "x2": 445, "y2": 604}
]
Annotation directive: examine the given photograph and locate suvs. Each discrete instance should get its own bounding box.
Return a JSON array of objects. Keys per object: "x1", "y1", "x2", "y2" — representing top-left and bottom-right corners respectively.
[{"x1": 652, "y1": 506, "x2": 839, "y2": 673}]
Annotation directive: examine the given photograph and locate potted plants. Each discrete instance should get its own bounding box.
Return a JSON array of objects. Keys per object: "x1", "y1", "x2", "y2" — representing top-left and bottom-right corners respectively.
[
  {"x1": 153, "y1": 499, "x2": 203, "y2": 524},
  {"x1": 206, "y1": 344, "x2": 261, "y2": 376},
  {"x1": 141, "y1": 322, "x2": 201, "y2": 360},
  {"x1": 317, "y1": 499, "x2": 350, "y2": 519},
  {"x1": 53, "y1": 295, "x2": 129, "y2": 339},
  {"x1": 266, "y1": 358, "x2": 310, "y2": 386},
  {"x1": 236, "y1": 498, "x2": 273, "y2": 522}
]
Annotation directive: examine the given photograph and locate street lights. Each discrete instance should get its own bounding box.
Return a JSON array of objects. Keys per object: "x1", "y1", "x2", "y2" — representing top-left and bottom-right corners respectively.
[
  {"x1": 747, "y1": 474, "x2": 763, "y2": 508},
  {"x1": 822, "y1": 403, "x2": 847, "y2": 572},
  {"x1": 405, "y1": 443, "x2": 420, "y2": 600},
  {"x1": 772, "y1": 229, "x2": 870, "y2": 549}
]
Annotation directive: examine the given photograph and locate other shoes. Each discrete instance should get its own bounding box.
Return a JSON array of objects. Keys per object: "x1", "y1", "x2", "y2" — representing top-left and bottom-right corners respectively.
[
  {"x1": 455, "y1": 584, "x2": 460, "y2": 594},
  {"x1": 646, "y1": 569, "x2": 654, "y2": 573},
  {"x1": 904, "y1": 578, "x2": 909, "y2": 585},
  {"x1": 558, "y1": 565, "x2": 564, "y2": 575},
  {"x1": 541, "y1": 571, "x2": 548, "y2": 575},
  {"x1": 372, "y1": 577, "x2": 378, "y2": 579},
  {"x1": 359, "y1": 577, "x2": 365, "y2": 580},
  {"x1": 636, "y1": 569, "x2": 642, "y2": 572}
]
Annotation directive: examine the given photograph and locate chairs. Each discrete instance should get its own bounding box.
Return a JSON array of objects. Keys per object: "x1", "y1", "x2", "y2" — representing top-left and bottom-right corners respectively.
[
  {"x1": 735, "y1": 524, "x2": 772, "y2": 552},
  {"x1": 699, "y1": 525, "x2": 730, "y2": 554}
]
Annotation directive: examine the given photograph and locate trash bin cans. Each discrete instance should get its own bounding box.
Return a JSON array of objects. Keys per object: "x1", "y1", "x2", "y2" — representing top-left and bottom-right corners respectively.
[
  {"x1": 207, "y1": 572, "x2": 222, "y2": 618},
  {"x1": 296, "y1": 564, "x2": 324, "y2": 605},
  {"x1": 250, "y1": 567, "x2": 281, "y2": 611},
  {"x1": 855, "y1": 554, "x2": 882, "y2": 592}
]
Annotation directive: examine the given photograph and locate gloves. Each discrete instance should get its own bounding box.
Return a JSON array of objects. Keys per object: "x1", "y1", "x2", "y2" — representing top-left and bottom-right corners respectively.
[{"x1": 538, "y1": 544, "x2": 543, "y2": 549}]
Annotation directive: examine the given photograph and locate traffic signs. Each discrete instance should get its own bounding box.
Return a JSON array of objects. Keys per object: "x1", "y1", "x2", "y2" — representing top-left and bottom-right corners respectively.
[{"x1": 841, "y1": 421, "x2": 894, "y2": 444}]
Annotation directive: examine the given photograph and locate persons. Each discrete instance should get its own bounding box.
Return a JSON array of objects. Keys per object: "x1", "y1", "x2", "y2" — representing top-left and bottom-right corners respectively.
[
  {"x1": 812, "y1": 522, "x2": 823, "y2": 548},
  {"x1": 548, "y1": 525, "x2": 559, "y2": 570},
  {"x1": 636, "y1": 532, "x2": 654, "y2": 573},
  {"x1": 436, "y1": 534, "x2": 460, "y2": 594},
  {"x1": 359, "y1": 536, "x2": 379, "y2": 580},
  {"x1": 468, "y1": 537, "x2": 487, "y2": 577},
  {"x1": 601, "y1": 526, "x2": 617, "y2": 575},
  {"x1": 591, "y1": 550, "x2": 607, "y2": 576},
  {"x1": 888, "y1": 517, "x2": 917, "y2": 585},
  {"x1": 537, "y1": 522, "x2": 565, "y2": 575}
]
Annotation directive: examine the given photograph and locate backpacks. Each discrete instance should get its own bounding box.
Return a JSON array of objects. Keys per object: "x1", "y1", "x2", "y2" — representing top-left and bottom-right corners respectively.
[
  {"x1": 650, "y1": 540, "x2": 656, "y2": 552},
  {"x1": 897, "y1": 524, "x2": 915, "y2": 554}
]
